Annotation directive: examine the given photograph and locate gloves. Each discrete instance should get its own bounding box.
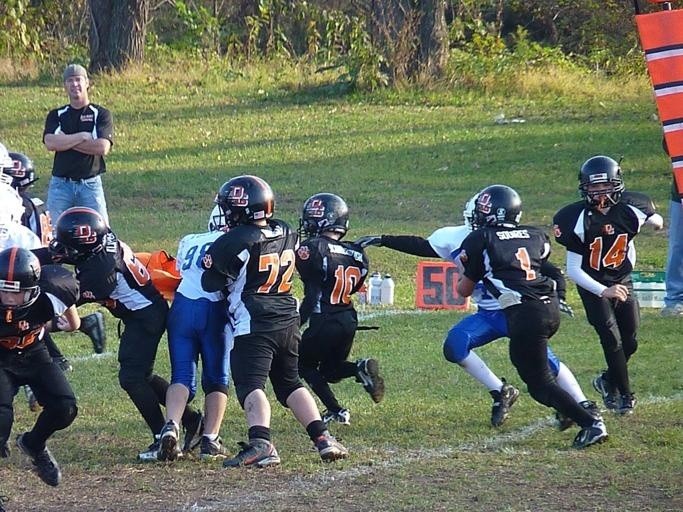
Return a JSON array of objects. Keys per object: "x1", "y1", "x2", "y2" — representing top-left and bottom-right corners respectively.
[{"x1": 354, "y1": 235, "x2": 381, "y2": 248}]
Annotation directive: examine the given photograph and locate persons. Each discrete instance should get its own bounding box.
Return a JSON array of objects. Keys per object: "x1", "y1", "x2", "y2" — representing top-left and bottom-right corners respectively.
[
  {"x1": 553, "y1": 155, "x2": 663, "y2": 414},
  {"x1": 662, "y1": 134, "x2": 683, "y2": 316},
  {"x1": 354, "y1": 193, "x2": 604, "y2": 432},
  {"x1": 42, "y1": 63, "x2": 113, "y2": 230},
  {"x1": 456, "y1": 185, "x2": 608, "y2": 448}
]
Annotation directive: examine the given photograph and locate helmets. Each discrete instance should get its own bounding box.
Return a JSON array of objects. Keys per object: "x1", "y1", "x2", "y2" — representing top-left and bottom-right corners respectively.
[
  {"x1": 48, "y1": 207, "x2": 108, "y2": 263},
  {"x1": 208, "y1": 175, "x2": 275, "y2": 233},
  {"x1": 297, "y1": 193, "x2": 349, "y2": 241},
  {"x1": 0, "y1": 247, "x2": 41, "y2": 323},
  {"x1": 0, "y1": 143, "x2": 40, "y2": 192},
  {"x1": 578, "y1": 155, "x2": 625, "y2": 208},
  {"x1": 463, "y1": 185, "x2": 522, "y2": 230}
]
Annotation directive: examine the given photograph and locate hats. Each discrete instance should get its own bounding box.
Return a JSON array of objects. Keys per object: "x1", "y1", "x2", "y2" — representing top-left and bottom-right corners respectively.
[{"x1": 64, "y1": 64, "x2": 86, "y2": 77}]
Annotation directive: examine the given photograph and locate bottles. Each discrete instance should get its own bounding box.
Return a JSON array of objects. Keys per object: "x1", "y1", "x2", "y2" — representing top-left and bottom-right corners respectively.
[{"x1": 358, "y1": 271, "x2": 394, "y2": 305}]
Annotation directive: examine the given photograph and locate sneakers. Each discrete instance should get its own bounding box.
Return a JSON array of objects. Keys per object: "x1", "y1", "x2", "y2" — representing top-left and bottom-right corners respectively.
[
  {"x1": 85, "y1": 313, "x2": 106, "y2": 353},
  {"x1": 489, "y1": 377, "x2": 519, "y2": 426},
  {"x1": 321, "y1": 408, "x2": 350, "y2": 425},
  {"x1": 316, "y1": 435, "x2": 349, "y2": 459},
  {"x1": 16, "y1": 355, "x2": 73, "y2": 486},
  {"x1": 356, "y1": 359, "x2": 385, "y2": 402},
  {"x1": 137, "y1": 409, "x2": 281, "y2": 468},
  {"x1": 556, "y1": 371, "x2": 637, "y2": 448}
]
